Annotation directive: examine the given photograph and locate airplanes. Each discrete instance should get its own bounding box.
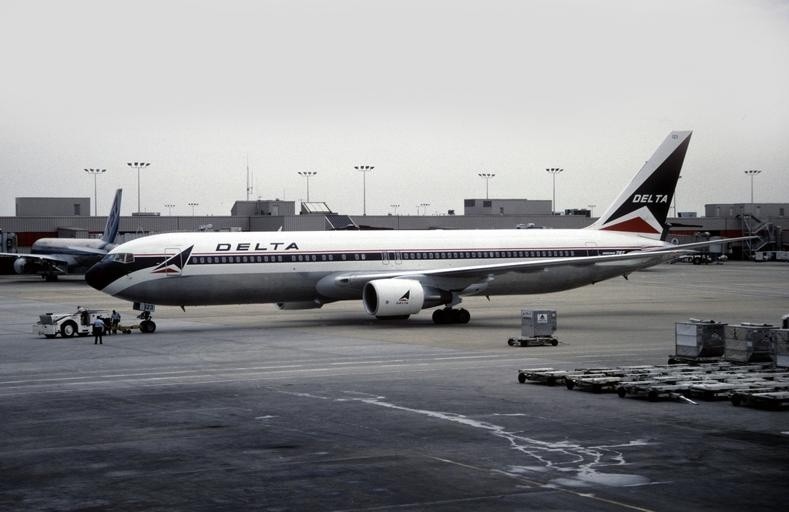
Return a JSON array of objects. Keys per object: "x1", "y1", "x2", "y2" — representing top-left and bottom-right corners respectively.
[
  {"x1": 83, "y1": 124, "x2": 693, "y2": 334},
  {"x1": 0, "y1": 188, "x2": 125, "y2": 283}
]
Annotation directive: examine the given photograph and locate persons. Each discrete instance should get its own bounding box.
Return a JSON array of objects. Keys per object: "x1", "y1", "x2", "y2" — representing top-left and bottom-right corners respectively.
[
  {"x1": 77, "y1": 306, "x2": 88, "y2": 325},
  {"x1": 93, "y1": 316, "x2": 107, "y2": 344},
  {"x1": 110, "y1": 310, "x2": 121, "y2": 335}
]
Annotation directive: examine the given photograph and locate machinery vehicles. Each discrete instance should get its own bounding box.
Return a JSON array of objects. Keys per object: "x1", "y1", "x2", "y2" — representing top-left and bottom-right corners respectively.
[{"x1": 30, "y1": 305, "x2": 114, "y2": 339}]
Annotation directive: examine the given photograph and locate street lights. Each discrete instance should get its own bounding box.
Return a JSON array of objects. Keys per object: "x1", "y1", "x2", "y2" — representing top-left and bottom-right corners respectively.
[
  {"x1": 296, "y1": 170, "x2": 318, "y2": 203},
  {"x1": 744, "y1": 168, "x2": 762, "y2": 262},
  {"x1": 126, "y1": 160, "x2": 153, "y2": 218},
  {"x1": 353, "y1": 165, "x2": 375, "y2": 217},
  {"x1": 162, "y1": 200, "x2": 201, "y2": 216},
  {"x1": 545, "y1": 166, "x2": 564, "y2": 216},
  {"x1": 477, "y1": 172, "x2": 496, "y2": 200},
  {"x1": 389, "y1": 201, "x2": 432, "y2": 216},
  {"x1": 83, "y1": 166, "x2": 108, "y2": 216}
]
